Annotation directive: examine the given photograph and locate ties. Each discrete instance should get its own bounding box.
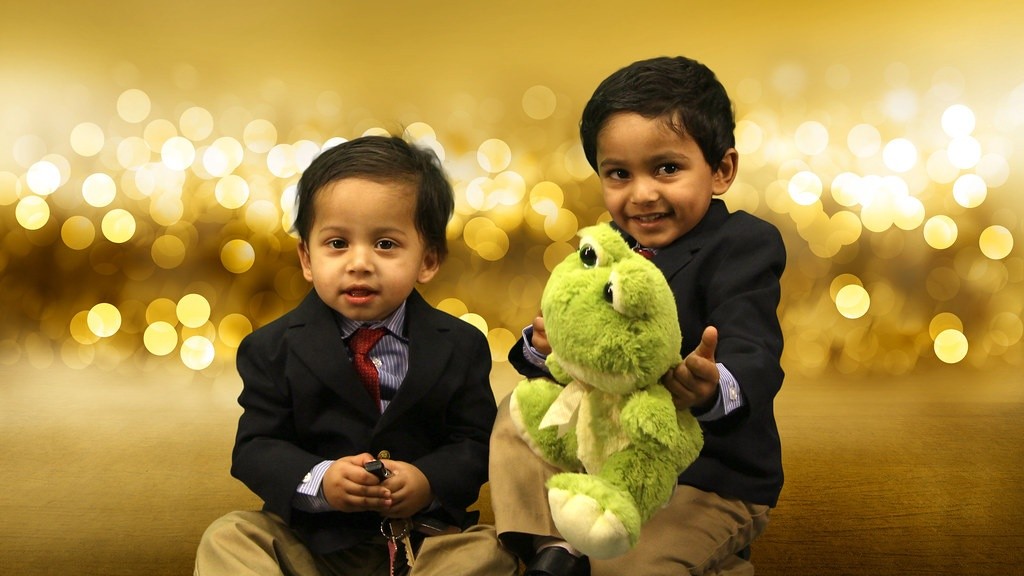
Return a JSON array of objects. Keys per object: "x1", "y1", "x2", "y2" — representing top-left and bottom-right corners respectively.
[
  {"x1": 343, "y1": 327, "x2": 390, "y2": 417},
  {"x1": 634, "y1": 248, "x2": 653, "y2": 263}
]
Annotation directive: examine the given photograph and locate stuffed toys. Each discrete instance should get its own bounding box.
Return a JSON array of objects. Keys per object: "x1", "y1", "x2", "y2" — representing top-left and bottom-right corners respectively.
[{"x1": 509, "y1": 220, "x2": 704, "y2": 558}]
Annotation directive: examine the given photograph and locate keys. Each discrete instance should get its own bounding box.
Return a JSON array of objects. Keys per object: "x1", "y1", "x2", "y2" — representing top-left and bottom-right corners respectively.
[{"x1": 361, "y1": 459, "x2": 463, "y2": 575}]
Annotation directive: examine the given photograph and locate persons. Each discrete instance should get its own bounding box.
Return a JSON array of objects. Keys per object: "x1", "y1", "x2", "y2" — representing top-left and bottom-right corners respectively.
[
  {"x1": 488, "y1": 55, "x2": 786, "y2": 574},
  {"x1": 191, "y1": 134, "x2": 517, "y2": 576}
]
